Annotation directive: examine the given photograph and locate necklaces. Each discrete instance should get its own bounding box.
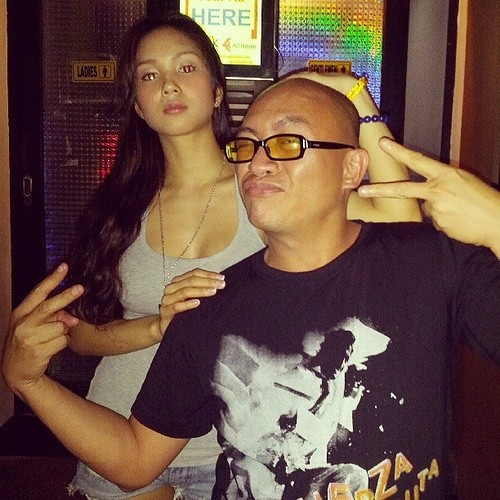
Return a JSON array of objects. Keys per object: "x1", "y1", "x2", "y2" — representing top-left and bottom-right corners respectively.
[{"x1": 158, "y1": 151, "x2": 226, "y2": 291}]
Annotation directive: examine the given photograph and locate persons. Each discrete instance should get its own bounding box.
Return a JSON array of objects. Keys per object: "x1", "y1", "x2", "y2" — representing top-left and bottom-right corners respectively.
[
  {"x1": 1, "y1": 77, "x2": 500, "y2": 500},
  {"x1": 66, "y1": 11, "x2": 423, "y2": 500}
]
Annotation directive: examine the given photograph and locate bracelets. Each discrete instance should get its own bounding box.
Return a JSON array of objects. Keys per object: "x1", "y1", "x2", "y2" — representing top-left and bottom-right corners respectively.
[
  {"x1": 344, "y1": 76, "x2": 367, "y2": 100},
  {"x1": 357, "y1": 115, "x2": 388, "y2": 124}
]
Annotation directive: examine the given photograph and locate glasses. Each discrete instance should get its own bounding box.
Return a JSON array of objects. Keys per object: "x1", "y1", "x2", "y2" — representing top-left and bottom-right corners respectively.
[{"x1": 225, "y1": 133, "x2": 355, "y2": 163}]
